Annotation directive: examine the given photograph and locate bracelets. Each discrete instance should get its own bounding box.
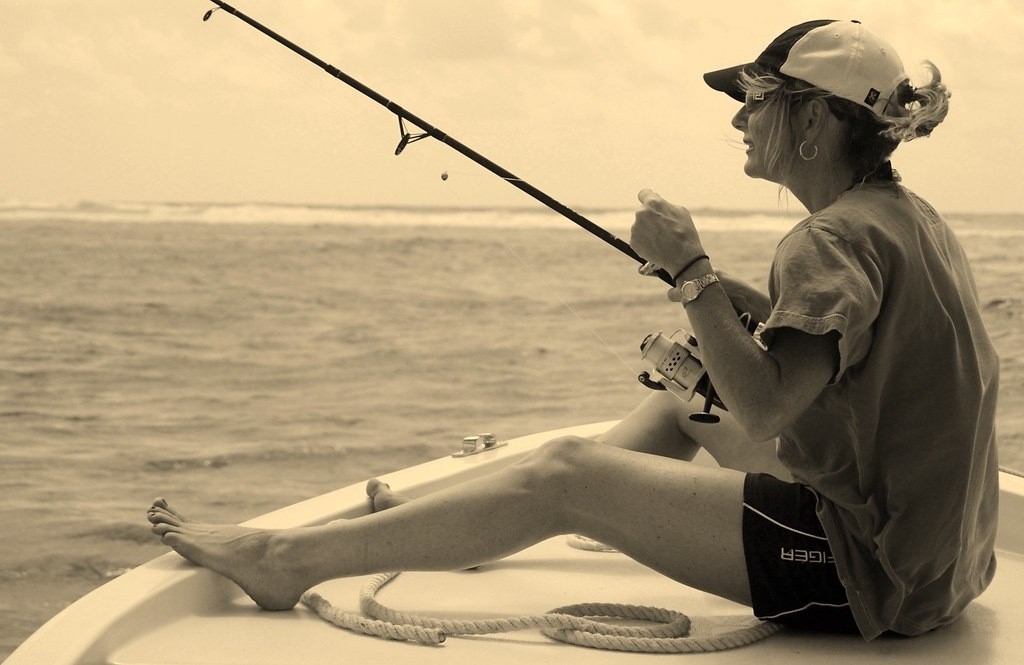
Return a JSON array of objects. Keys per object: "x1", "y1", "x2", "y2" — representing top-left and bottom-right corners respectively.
[{"x1": 672, "y1": 254, "x2": 709, "y2": 281}]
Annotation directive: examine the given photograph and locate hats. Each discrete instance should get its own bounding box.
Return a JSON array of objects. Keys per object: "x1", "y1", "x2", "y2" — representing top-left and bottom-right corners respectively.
[{"x1": 703, "y1": 19, "x2": 910, "y2": 118}]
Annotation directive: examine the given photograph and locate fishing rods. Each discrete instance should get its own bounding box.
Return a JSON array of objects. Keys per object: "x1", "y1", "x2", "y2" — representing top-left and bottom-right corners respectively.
[{"x1": 194, "y1": 0, "x2": 751, "y2": 424}]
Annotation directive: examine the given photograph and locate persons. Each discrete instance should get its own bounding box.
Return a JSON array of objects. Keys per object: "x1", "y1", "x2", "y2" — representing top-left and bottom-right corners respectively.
[{"x1": 144, "y1": 17, "x2": 1000, "y2": 642}]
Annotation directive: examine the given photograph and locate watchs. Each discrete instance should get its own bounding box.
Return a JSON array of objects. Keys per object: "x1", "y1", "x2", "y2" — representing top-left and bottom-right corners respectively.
[{"x1": 681, "y1": 274, "x2": 718, "y2": 308}]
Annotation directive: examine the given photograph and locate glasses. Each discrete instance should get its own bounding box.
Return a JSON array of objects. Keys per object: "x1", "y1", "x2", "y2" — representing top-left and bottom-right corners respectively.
[{"x1": 745, "y1": 87, "x2": 784, "y2": 112}]
